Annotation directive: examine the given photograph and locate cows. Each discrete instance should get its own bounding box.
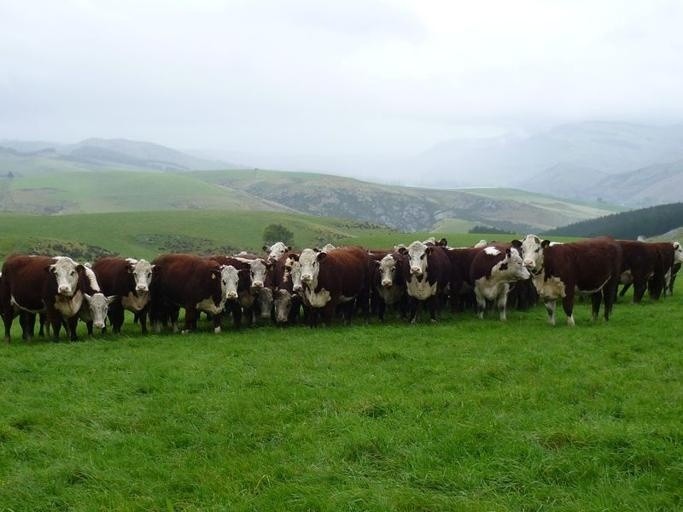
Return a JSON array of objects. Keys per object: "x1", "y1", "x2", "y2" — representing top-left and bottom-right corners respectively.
[{"x1": 0, "y1": 233, "x2": 683, "y2": 345}]
